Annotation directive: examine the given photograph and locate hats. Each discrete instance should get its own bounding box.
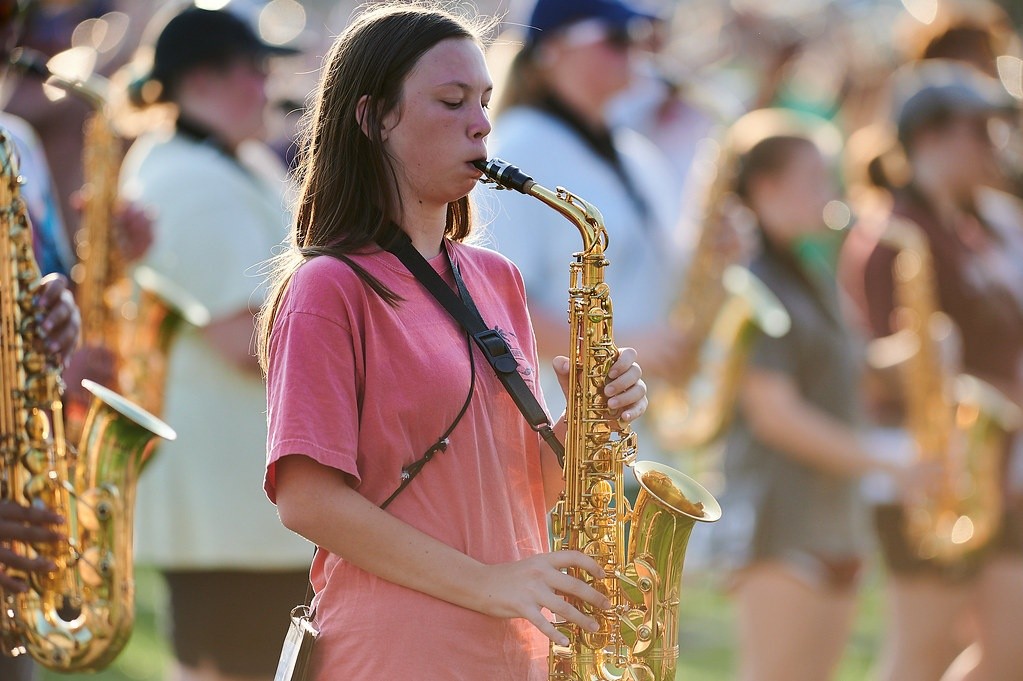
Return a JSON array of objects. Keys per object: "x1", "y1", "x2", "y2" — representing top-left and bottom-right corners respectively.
[
  {"x1": 528, "y1": 0, "x2": 665, "y2": 48},
  {"x1": 154, "y1": 8, "x2": 302, "y2": 76},
  {"x1": 901, "y1": 68, "x2": 1021, "y2": 154}
]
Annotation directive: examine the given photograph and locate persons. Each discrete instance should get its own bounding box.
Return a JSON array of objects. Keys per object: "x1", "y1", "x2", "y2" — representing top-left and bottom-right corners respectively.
[
  {"x1": 0, "y1": 0, "x2": 316, "y2": 681},
  {"x1": 686, "y1": 109, "x2": 949, "y2": 681},
  {"x1": 471, "y1": 0, "x2": 692, "y2": 488},
  {"x1": 257, "y1": 9, "x2": 649, "y2": 681},
  {"x1": 837, "y1": 22, "x2": 1023, "y2": 681}
]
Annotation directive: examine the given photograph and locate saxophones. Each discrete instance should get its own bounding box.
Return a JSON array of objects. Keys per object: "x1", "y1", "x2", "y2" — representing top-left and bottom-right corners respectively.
[
  {"x1": 1, "y1": 120, "x2": 176, "y2": 670},
  {"x1": 470, "y1": 157, "x2": 725, "y2": 681},
  {"x1": 854, "y1": 194, "x2": 1018, "y2": 567},
  {"x1": 69, "y1": 81, "x2": 211, "y2": 417},
  {"x1": 625, "y1": 76, "x2": 791, "y2": 452}
]
